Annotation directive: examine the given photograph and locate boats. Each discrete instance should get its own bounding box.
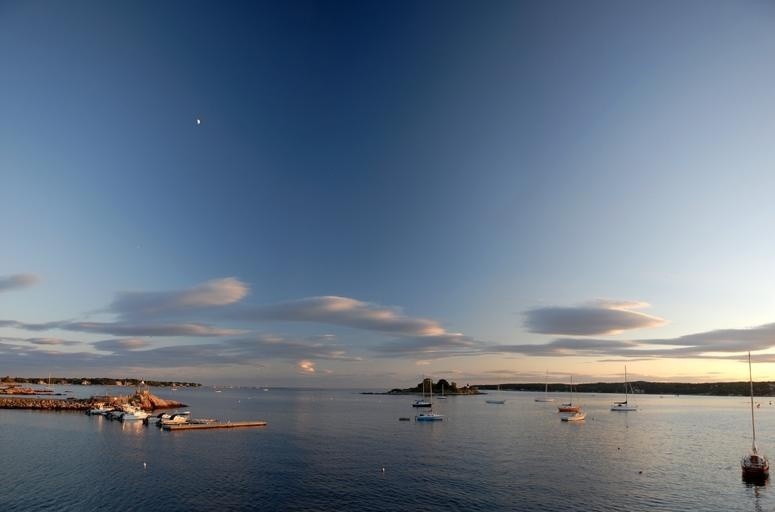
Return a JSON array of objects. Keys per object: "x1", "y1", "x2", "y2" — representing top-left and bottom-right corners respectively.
[
  {"x1": 413, "y1": 374, "x2": 447, "y2": 422},
  {"x1": 610, "y1": 364, "x2": 638, "y2": 411},
  {"x1": 535, "y1": 376, "x2": 589, "y2": 422},
  {"x1": 485, "y1": 399, "x2": 505, "y2": 404},
  {"x1": 741, "y1": 353, "x2": 770, "y2": 483},
  {"x1": 86, "y1": 407, "x2": 266, "y2": 431}
]
